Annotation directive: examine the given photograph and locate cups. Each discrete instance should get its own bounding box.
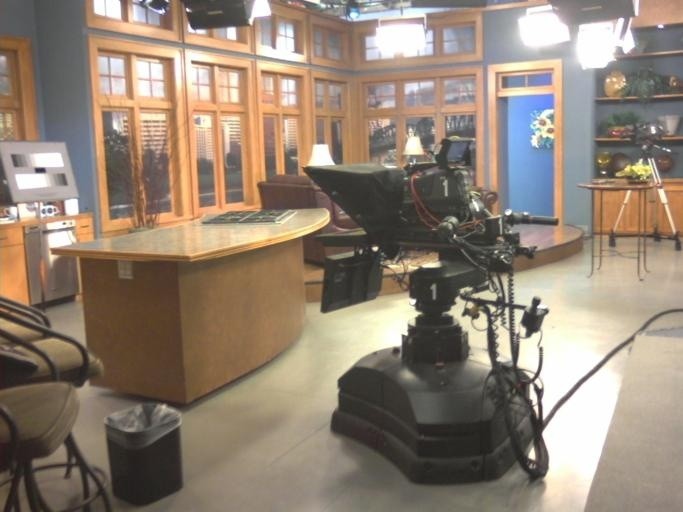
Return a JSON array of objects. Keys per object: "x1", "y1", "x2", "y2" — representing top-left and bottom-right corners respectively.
[{"x1": 3, "y1": 206, "x2": 18, "y2": 217}]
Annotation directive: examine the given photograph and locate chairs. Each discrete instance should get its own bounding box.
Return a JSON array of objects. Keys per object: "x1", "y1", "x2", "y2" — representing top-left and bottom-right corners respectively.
[{"x1": 0, "y1": 296, "x2": 112, "y2": 512}]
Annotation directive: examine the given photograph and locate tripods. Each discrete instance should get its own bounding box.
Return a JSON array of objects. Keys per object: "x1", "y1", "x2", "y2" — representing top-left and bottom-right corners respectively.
[{"x1": 609, "y1": 144, "x2": 681, "y2": 250}]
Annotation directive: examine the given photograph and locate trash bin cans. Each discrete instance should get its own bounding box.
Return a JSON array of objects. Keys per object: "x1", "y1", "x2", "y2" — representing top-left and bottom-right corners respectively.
[{"x1": 103, "y1": 403, "x2": 182, "y2": 506}]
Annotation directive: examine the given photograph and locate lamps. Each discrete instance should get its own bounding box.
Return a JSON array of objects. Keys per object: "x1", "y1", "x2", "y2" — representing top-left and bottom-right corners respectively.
[{"x1": 305, "y1": 134, "x2": 427, "y2": 169}]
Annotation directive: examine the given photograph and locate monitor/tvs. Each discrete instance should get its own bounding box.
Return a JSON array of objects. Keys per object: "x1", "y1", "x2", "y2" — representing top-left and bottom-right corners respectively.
[
  {"x1": 302, "y1": 162, "x2": 405, "y2": 259},
  {"x1": 320, "y1": 245, "x2": 383, "y2": 313}
]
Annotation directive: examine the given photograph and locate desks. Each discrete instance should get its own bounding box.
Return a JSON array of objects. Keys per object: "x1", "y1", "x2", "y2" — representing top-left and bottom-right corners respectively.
[
  {"x1": 52, "y1": 205, "x2": 332, "y2": 406},
  {"x1": 578, "y1": 182, "x2": 663, "y2": 281}
]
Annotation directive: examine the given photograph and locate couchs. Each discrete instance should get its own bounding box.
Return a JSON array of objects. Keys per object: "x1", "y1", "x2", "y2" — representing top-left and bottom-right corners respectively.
[{"x1": 257, "y1": 175, "x2": 362, "y2": 265}]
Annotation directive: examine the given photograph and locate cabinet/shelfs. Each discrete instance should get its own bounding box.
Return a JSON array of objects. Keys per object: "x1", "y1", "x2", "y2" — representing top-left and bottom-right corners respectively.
[
  {"x1": 587, "y1": 0, "x2": 683, "y2": 235},
  {"x1": 0, "y1": 210, "x2": 95, "y2": 303}
]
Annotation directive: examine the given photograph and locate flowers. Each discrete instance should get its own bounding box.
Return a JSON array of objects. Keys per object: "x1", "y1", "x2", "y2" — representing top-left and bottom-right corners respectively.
[{"x1": 617, "y1": 160, "x2": 652, "y2": 184}]
